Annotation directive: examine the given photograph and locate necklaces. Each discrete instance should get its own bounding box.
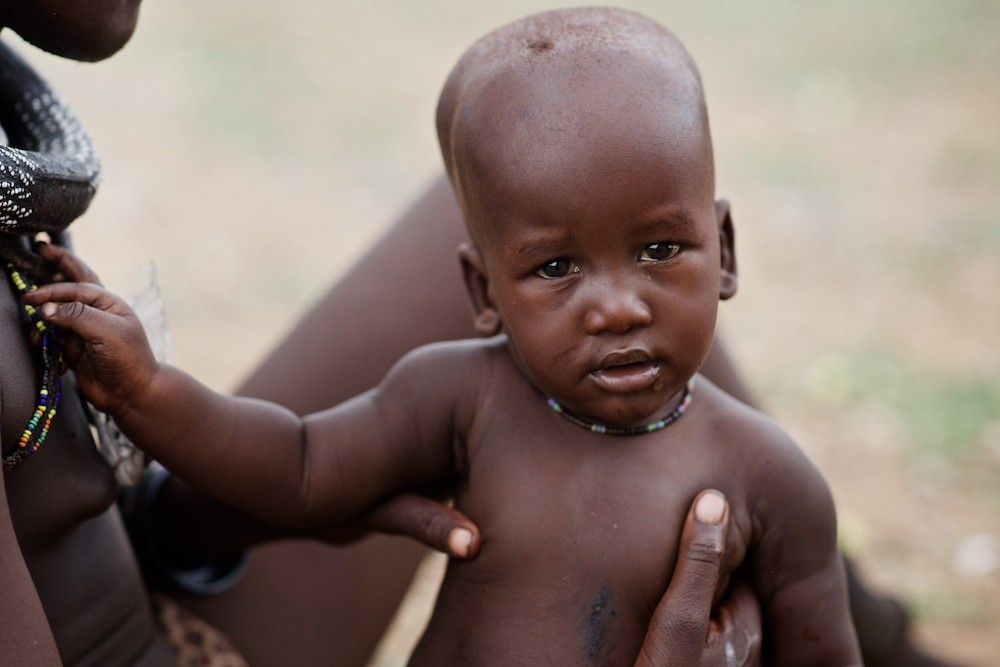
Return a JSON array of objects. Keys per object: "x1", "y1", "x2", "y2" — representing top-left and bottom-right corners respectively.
[
  {"x1": 546, "y1": 380, "x2": 694, "y2": 437},
  {"x1": 0, "y1": 37, "x2": 101, "y2": 470}
]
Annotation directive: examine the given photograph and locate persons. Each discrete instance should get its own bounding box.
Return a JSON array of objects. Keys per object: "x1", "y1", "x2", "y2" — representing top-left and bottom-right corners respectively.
[
  {"x1": 0, "y1": 0, "x2": 907, "y2": 667},
  {"x1": 28, "y1": 7, "x2": 864, "y2": 667}
]
